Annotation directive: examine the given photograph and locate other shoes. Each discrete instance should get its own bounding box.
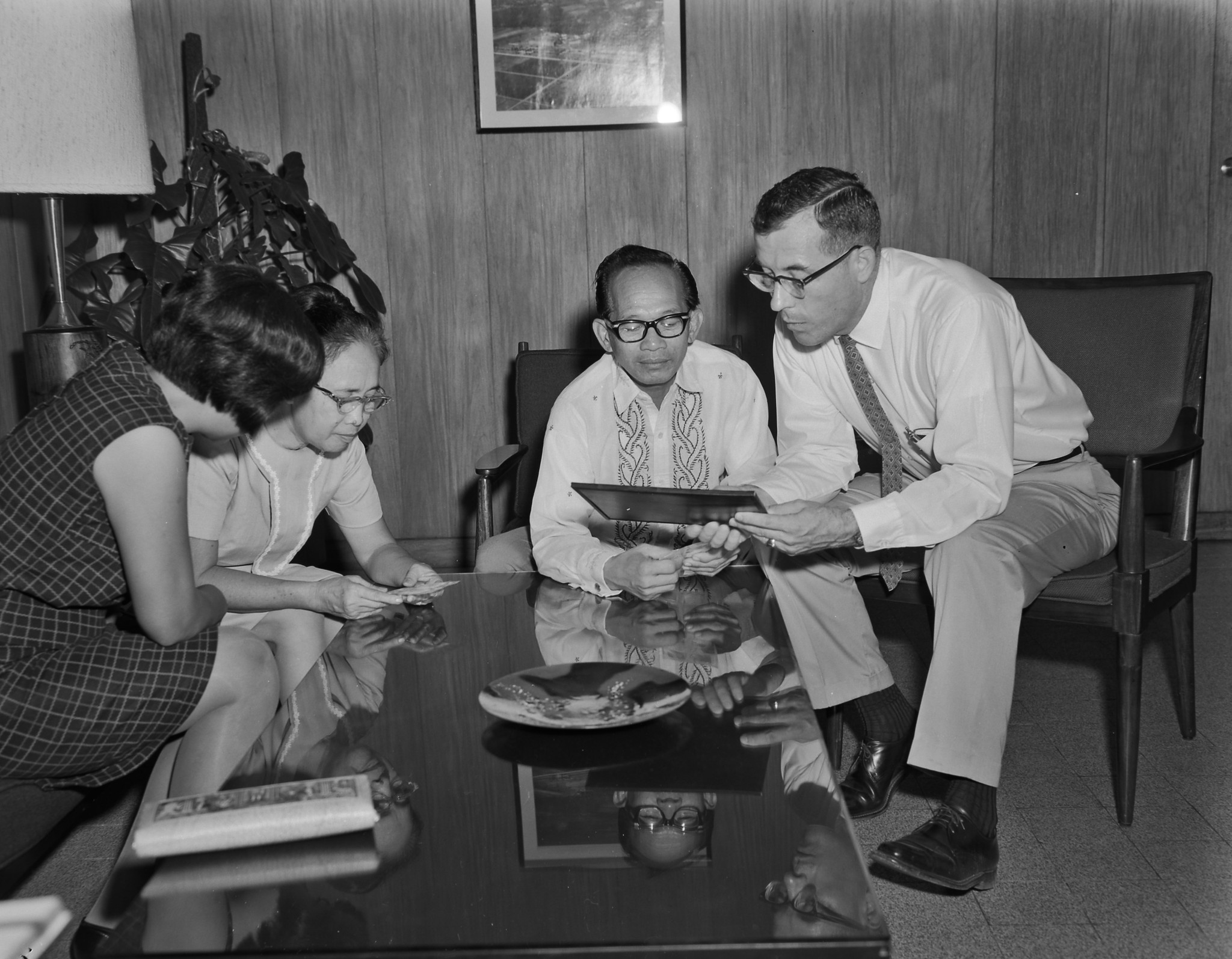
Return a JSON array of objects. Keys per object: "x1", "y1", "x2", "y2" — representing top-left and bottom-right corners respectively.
[{"x1": 71, "y1": 917, "x2": 114, "y2": 959}]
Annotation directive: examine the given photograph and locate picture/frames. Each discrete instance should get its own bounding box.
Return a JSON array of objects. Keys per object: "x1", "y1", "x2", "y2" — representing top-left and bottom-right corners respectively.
[
  {"x1": 515, "y1": 761, "x2": 714, "y2": 875},
  {"x1": 469, "y1": 0, "x2": 688, "y2": 135},
  {"x1": 570, "y1": 482, "x2": 770, "y2": 526}
]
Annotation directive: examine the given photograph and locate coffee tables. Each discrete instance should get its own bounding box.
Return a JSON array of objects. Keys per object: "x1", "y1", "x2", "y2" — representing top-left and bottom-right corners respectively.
[{"x1": 96, "y1": 566, "x2": 890, "y2": 959}]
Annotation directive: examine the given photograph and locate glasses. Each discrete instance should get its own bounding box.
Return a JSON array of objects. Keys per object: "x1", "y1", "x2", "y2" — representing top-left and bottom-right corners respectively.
[
  {"x1": 603, "y1": 307, "x2": 694, "y2": 344},
  {"x1": 757, "y1": 879, "x2": 870, "y2": 932},
  {"x1": 741, "y1": 245, "x2": 863, "y2": 299},
  {"x1": 371, "y1": 778, "x2": 420, "y2": 815},
  {"x1": 314, "y1": 383, "x2": 392, "y2": 415},
  {"x1": 624, "y1": 805, "x2": 705, "y2": 832}
]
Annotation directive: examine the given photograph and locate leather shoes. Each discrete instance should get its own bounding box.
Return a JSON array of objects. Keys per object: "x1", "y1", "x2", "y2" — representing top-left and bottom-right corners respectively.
[
  {"x1": 838, "y1": 728, "x2": 914, "y2": 821},
  {"x1": 869, "y1": 805, "x2": 1000, "y2": 891}
]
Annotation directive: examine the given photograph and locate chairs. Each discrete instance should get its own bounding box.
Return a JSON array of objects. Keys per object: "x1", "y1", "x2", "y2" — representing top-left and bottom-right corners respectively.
[
  {"x1": 475, "y1": 335, "x2": 745, "y2": 542},
  {"x1": 824, "y1": 271, "x2": 1214, "y2": 823}
]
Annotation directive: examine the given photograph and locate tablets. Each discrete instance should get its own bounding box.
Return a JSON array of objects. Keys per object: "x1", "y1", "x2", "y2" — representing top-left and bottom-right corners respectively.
[{"x1": 572, "y1": 482, "x2": 768, "y2": 525}]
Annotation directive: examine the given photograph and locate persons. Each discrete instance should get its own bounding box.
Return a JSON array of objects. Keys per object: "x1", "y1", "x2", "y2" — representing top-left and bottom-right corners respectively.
[
  {"x1": 534, "y1": 578, "x2": 803, "y2": 873},
  {"x1": 470, "y1": 244, "x2": 777, "y2": 599},
  {"x1": 225, "y1": 605, "x2": 452, "y2": 892},
  {"x1": 0, "y1": 267, "x2": 326, "y2": 805},
  {"x1": 128, "y1": 889, "x2": 370, "y2": 954},
  {"x1": 684, "y1": 168, "x2": 1124, "y2": 891},
  {"x1": 690, "y1": 646, "x2": 892, "y2": 940},
  {"x1": 182, "y1": 285, "x2": 444, "y2": 704}
]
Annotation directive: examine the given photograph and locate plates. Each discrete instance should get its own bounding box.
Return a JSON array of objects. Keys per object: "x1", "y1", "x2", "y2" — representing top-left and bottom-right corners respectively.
[{"x1": 478, "y1": 661, "x2": 692, "y2": 728}]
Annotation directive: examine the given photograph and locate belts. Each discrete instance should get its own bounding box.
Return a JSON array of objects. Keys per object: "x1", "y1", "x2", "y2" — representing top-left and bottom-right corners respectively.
[{"x1": 1032, "y1": 442, "x2": 1088, "y2": 468}]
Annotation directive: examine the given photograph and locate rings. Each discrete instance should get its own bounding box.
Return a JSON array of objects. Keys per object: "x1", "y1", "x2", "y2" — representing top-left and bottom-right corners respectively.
[
  {"x1": 772, "y1": 699, "x2": 779, "y2": 710},
  {"x1": 766, "y1": 539, "x2": 776, "y2": 548}
]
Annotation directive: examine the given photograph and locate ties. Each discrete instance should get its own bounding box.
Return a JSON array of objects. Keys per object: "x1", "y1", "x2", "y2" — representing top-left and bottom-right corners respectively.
[{"x1": 838, "y1": 335, "x2": 902, "y2": 593}]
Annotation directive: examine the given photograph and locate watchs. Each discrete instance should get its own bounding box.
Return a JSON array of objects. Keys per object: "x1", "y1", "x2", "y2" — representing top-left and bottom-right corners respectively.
[{"x1": 853, "y1": 530, "x2": 864, "y2": 548}]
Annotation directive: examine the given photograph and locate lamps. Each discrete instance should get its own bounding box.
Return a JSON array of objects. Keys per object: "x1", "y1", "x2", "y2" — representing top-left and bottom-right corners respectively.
[{"x1": 1, "y1": 1, "x2": 155, "y2": 407}]
